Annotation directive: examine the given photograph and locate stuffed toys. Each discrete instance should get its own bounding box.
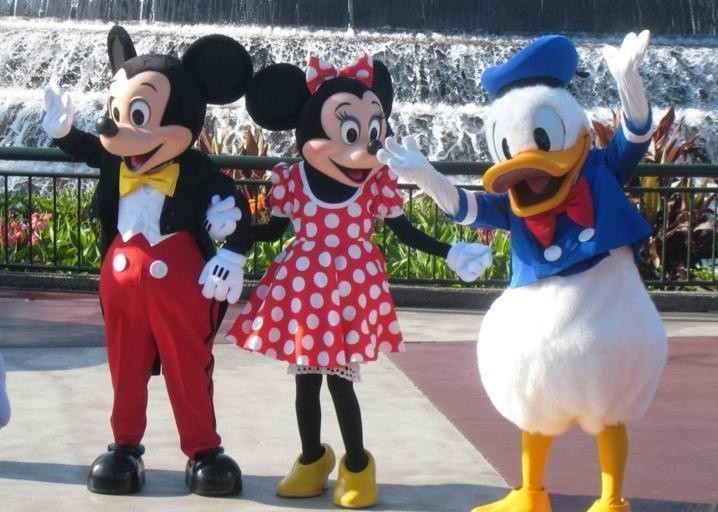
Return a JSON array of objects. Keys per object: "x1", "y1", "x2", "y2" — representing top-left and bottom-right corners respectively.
[
  {"x1": 42, "y1": 22, "x2": 254, "y2": 497},
  {"x1": 198, "y1": 52, "x2": 495, "y2": 509},
  {"x1": 377, "y1": 29, "x2": 672, "y2": 512}
]
